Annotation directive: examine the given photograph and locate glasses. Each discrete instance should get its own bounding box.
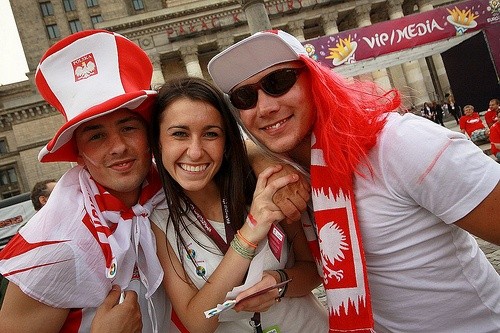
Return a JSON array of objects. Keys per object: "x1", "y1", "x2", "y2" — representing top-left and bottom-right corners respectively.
[{"x1": 230, "y1": 67, "x2": 306, "y2": 109}]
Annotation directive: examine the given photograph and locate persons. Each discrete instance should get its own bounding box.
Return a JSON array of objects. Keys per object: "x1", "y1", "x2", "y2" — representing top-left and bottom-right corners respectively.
[
  {"x1": 459, "y1": 105, "x2": 490, "y2": 146},
  {"x1": 484, "y1": 99, "x2": 500, "y2": 128},
  {"x1": 404, "y1": 92, "x2": 462, "y2": 127},
  {"x1": 489, "y1": 108, "x2": 500, "y2": 164},
  {"x1": 0, "y1": 28, "x2": 187, "y2": 333},
  {"x1": 18, "y1": 178, "x2": 60, "y2": 235},
  {"x1": 208, "y1": 28, "x2": 500, "y2": 333},
  {"x1": 145, "y1": 77, "x2": 330, "y2": 333}
]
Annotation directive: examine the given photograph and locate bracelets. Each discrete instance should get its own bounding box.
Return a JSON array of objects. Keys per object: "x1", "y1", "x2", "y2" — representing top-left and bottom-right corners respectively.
[
  {"x1": 273, "y1": 269, "x2": 289, "y2": 302},
  {"x1": 229, "y1": 228, "x2": 259, "y2": 261}
]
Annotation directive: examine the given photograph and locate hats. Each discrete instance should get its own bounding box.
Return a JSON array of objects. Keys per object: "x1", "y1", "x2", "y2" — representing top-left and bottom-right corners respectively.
[
  {"x1": 35, "y1": 29, "x2": 159, "y2": 162},
  {"x1": 207, "y1": 29, "x2": 310, "y2": 92}
]
